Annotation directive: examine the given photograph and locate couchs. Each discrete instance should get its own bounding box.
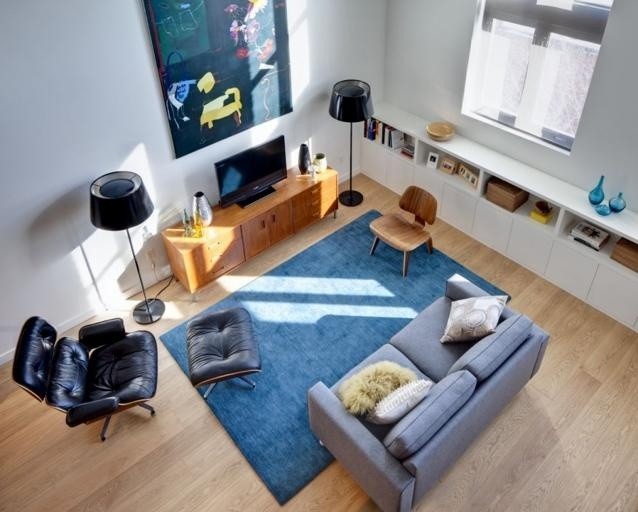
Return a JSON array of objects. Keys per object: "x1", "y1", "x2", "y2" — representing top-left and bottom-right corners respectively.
[{"x1": 308, "y1": 273, "x2": 551, "y2": 512}]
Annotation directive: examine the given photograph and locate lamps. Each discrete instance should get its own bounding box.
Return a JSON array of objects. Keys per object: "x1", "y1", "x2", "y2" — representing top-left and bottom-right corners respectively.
[
  {"x1": 89, "y1": 171, "x2": 166, "y2": 324},
  {"x1": 329, "y1": 79, "x2": 374, "y2": 207}
]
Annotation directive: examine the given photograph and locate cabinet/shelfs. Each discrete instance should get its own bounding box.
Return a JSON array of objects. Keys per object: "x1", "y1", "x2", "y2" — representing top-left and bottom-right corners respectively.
[
  {"x1": 161, "y1": 162, "x2": 341, "y2": 295},
  {"x1": 360, "y1": 103, "x2": 637, "y2": 333}
]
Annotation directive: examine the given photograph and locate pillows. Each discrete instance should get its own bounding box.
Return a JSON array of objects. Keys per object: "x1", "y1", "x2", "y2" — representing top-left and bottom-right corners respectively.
[
  {"x1": 383, "y1": 369, "x2": 476, "y2": 459},
  {"x1": 441, "y1": 295, "x2": 508, "y2": 345},
  {"x1": 365, "y1": 381, "x2": 431, "y2": 425},
  {"x1": 446, "y1": 313, "x2": 532, "y2": 380},
  {"x1": 338, "y1": 361, "x2": 418, "y2": 416}
]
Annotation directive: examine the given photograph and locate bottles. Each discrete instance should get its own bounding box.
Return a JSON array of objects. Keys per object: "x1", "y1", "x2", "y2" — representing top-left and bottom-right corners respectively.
[
  {"x1": 182, "y1": 208, "x2": 192, "y2": 237},
  {"x1": 192, "y1": 211, "x2": 205, "y2": 238}
]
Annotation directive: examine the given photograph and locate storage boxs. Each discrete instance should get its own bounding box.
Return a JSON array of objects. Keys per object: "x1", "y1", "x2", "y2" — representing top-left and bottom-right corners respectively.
[
  {"x1": 611, "y1": 239, "x2": 638, "y2": 271},
  {"x1": 487, "y1": 181, "x2": 529, "y2": 214}
]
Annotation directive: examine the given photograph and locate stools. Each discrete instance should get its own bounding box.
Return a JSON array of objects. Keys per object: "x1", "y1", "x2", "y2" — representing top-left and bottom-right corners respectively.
[{"x1": 185, "y1": 306, "x2": 263, "y2": 398}]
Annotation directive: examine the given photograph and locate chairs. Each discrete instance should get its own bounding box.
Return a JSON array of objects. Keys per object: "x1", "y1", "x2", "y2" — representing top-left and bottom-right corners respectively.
[
  {"x1": 369, "y1": 186, "x2": 437, "y2": 274},
  {"x1": 12, "y1": 316, "x2": 158, "y2": 441}
]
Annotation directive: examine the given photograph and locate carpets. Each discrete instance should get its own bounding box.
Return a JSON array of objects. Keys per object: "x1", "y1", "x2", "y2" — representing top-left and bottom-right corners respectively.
[{"x1": 159, "y1": 210, "x2": 512, "y2": 506}]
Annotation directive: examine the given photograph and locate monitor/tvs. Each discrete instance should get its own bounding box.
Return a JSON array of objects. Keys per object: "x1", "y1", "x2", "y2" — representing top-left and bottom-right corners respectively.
[{"x1": 214, "y1": 134, "x2": 287, "y2": 209}]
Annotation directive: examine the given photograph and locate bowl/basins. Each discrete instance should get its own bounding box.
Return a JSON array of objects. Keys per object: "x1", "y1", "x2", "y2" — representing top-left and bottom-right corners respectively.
[{"x1": 426, "y1": 122, "x2": 454, "y2": 140}]
